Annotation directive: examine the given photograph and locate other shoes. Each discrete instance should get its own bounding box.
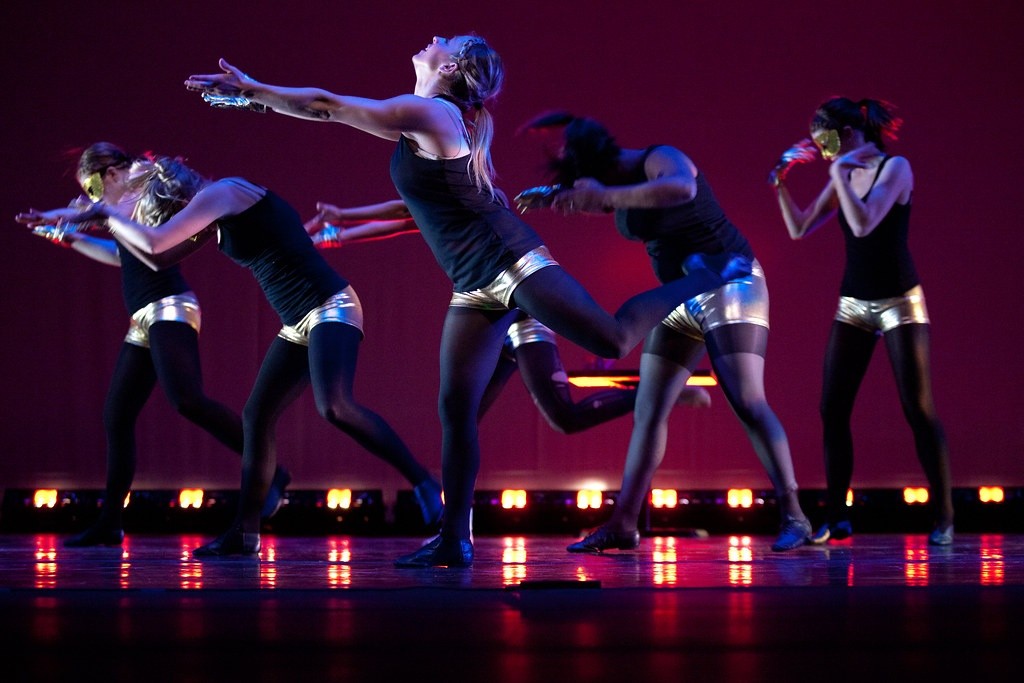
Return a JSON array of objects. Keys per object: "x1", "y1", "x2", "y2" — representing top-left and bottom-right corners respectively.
[
  {"x1": 812, "y1": 520, "x2": 852, "y2": 543},
  {"x1": 567, "y1": 525, "x2": 640, "y2": 553},
  {"x1": 193, "y1": 529, "x2": 260, "y2": 556},
  {"x1": 261, "y1": 464, "x2": 291, "y2": 517},
  {"x1": 772, "y1": 521, "x2": 812, "y2": 552},
  {"x1": 413, "y1": 476, "x2": 444, "y2": 526},
  {"x1": 682, "y1": 253, "x2": 751, "y2": 285},
  {"x1": 393, "y1": 536, "x2": 474, "y2": 569},
  {"x1": 63, "y1": 526, "x2": 124, "y2": 547},
  {"x1": 928, "y1": 524, "x2": 953, "y2": 545}
]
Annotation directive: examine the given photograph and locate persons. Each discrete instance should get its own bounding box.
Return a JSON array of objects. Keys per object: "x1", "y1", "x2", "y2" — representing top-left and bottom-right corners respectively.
[
  {"x1": 14, "y1": 140, "x2": 445, "y2": 560},
  {"x1": 183, "y1": 33, "x2": 754, "y2": 566},
  {"x1": 766, "y1": 90, "x2": 955, "y2": 552},
  {"x1": 514, "y1": 109, "x2": 813, "y2": 554}
]
